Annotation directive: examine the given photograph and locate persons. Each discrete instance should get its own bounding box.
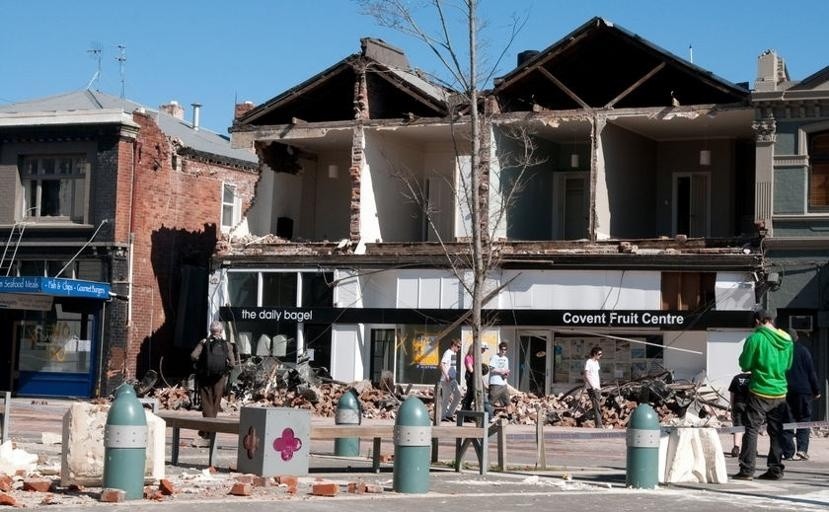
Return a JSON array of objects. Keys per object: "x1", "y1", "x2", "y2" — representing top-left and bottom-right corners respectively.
[
  {"x1": 191, "y1": 322, "x2": 236, "y2": 441},
  {"x1": 728, "y1": 371, "x2": 758, "y2": 457},
  {"x1": 490, "y1": 341, "x2": 515, "y2": 424},
  {"x1": 441, "y1": 338, "x2": 463, "y2": 423},
  {"x1": 789, "y1": 328, "x2": 820, "y2": 460},
  {"x1": 577, "y1": 348, "x2": 607, "y2": 428},
  {"x1": 734, "y1": 307, "x2": 792, "y2": 480},
  {"x1": 464, "y1": 339, "x2": 490, "y2": 427}
]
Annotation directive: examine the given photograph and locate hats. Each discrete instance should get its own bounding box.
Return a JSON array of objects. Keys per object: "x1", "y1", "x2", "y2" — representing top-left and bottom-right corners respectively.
[{"x1": 481, "y1": 341, "x2": 489, "y2": 349}]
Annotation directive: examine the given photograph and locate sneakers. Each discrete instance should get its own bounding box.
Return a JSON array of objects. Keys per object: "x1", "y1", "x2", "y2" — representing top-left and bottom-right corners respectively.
[
  {"x1": 796, "y1": 451, "x2": 810, "y2": 460},
  {"x1": 758, "y1": 473, "x2": 784, "y2": 480},
  {"x1": 733, "y1": 472, "x2": 754, "y2": 481}
]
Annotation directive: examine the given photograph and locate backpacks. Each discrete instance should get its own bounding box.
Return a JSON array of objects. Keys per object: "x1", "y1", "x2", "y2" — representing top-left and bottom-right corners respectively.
[{"x1": 197, "y1": 336, "x2": 230, "y2": 385}]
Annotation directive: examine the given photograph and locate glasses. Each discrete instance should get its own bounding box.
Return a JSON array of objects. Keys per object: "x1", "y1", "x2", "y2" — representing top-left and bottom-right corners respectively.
[
  {"x1": 499, "y1": 348, "x2": 509, "y2": 351},
  {"x1": 594, "y1": 353, "x2": 604, "y2": 356},
  {"x1": 456, "y1": 345, "x2": 462, "y2": 349}
]
